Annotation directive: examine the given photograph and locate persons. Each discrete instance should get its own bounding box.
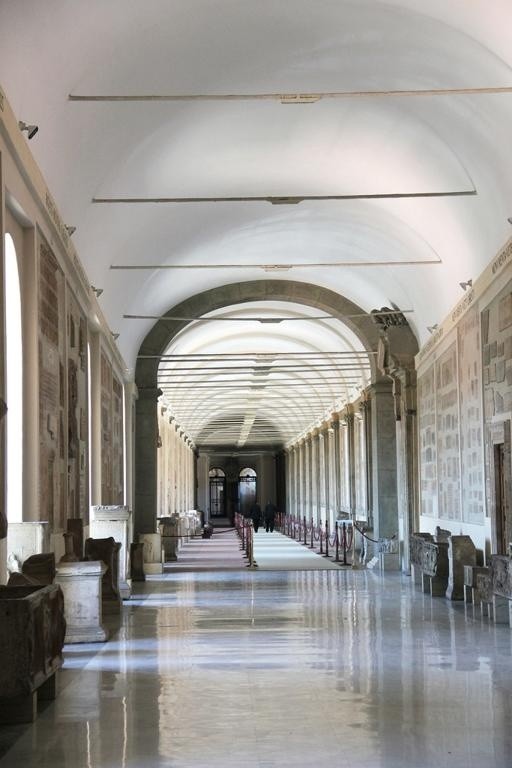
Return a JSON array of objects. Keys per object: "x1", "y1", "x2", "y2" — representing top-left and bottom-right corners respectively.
[
  {"x1": 251, "y1": 500, "x2": 262, "y2": 533},
  {"x1": 263, "y1": 498, "x2": 278, "y2": 533}
]
